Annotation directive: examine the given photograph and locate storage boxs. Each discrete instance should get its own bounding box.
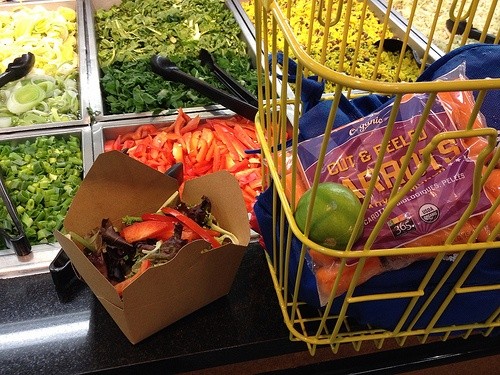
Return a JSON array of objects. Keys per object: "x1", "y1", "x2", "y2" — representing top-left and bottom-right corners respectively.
[
  {"x1": 52, "y1": 150, "x2": 253, "y2": 346},
  {"x1": 253, "y1": 1, "x2": 500, "y2": 355}
]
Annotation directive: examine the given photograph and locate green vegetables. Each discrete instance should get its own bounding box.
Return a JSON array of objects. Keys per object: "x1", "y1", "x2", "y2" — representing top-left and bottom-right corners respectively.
[{"x1": 93, "y1": 0, "x2": 267, "y2": 115}]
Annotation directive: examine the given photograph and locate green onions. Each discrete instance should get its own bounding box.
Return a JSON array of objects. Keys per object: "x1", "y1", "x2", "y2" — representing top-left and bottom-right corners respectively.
[{"x1": 0, "y1": 67, "x2": 83, "y2": 248}]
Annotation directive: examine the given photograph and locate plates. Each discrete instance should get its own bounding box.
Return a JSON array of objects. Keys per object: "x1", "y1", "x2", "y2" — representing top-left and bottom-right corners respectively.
[
  {"x1": 88, "y1": 103, "x2": 300, "y2": 244},
  {"x1": 377, "y1": 0, "x2": 500, "y2": 58},
  {"x1": 83, "y1": 0, "x2": 291, "y2": 124},
  {"x1": 233, "y1": 0, "x2": 449, "y2": 99},
  {"x1": 0, "y1": 1, "x2": 93, "y2": 133},
  {"x1": 0, "y1": 126, "x2": 92, "y2": 253}
]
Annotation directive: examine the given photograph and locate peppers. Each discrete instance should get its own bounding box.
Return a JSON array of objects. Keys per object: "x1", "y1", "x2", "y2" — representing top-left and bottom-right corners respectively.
[{"x1": 106, "y1": 114, "x2": 293, "y2": 240}]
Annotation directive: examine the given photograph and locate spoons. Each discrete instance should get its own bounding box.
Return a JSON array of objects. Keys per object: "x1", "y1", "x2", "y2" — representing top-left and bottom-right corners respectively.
[{"x1": 374, "y1": 37, "x2": 442, "y2": 69}]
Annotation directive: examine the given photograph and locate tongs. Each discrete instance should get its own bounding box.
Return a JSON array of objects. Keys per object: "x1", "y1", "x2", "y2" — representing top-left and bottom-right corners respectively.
[
  {"x1": 0, "y1": 181, "x2": 31, "y2": 258},
  {"x1": 146, "y1": 47, "x2": 272, "y2": 124},
  {"x1": 444, "y1": 20, "x2": 500, "y2": 46},
  {"x1": 0, "y1": 52, "x2": 35, "y2": 89}
]
